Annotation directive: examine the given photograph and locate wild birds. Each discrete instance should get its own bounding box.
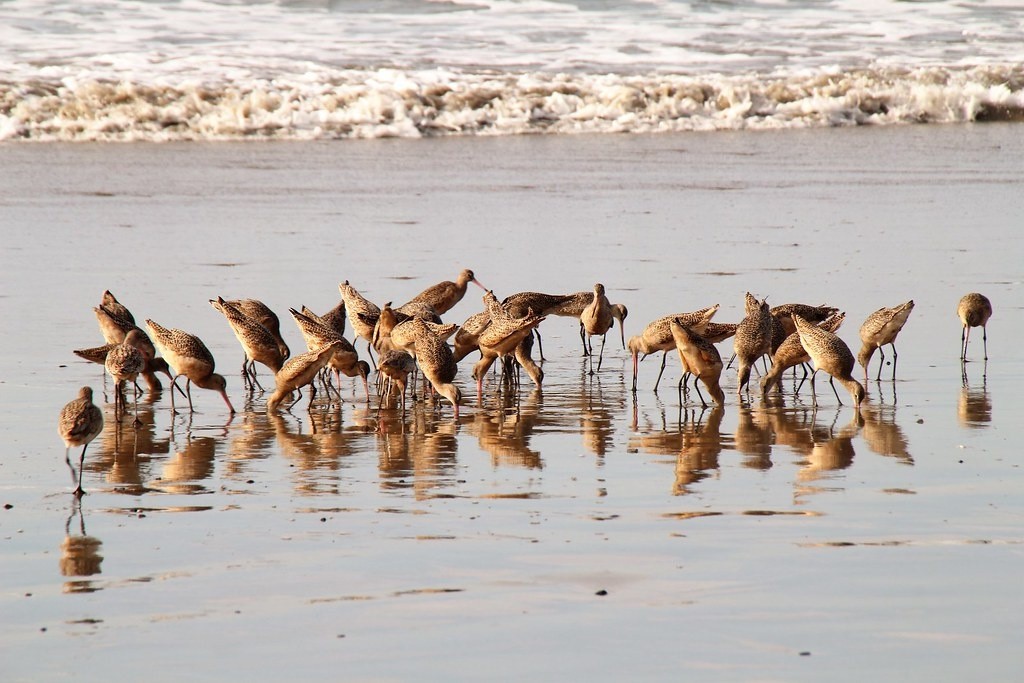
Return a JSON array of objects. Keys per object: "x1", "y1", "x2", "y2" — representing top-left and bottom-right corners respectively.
[
  {"x1": 791, "y1": 313, "x2": 865, "y2": 407},
  {"x1": 669, "y1": 318, "x2": 725, "y2": 408},
  {"x1": 551, "y1": 291, "x2": 628, "y2": 356},
  {"x1": 453, "y1": 290, "x2": 574, "y2": 386},
  {"x1": 393, "y1": 268, "x2": 488, "y2": 315},
  {"x1": 734, "y1": 290, "x2": 839, "y2": 393},
  {"x1": 957, "y1": 292, "x2": 992, "y2": 363},
  {"x1": 287, "y1": 301, "x2": 370, "y2": 401},
  {"x1": 209, "y1": 295, "x2": 289, "y2": 392},
  {"x1": 857, "y1": 300, "x2": 915, "y2": 381},
  {"x1": 759, "y1": 312, "x2": 845, "y2": 401},
  {"x1": 73, "y1": 290, "x2": 186, "y2": 426},
  {"x1": 470, "y1": 306, "x2": 546, "y2": 407},
  {"x1": 144, "y1": 318, "x2": 236, "y2": 415},
  {"x1": 412, "y1": 315, "x2": 461, "y2": 420},
  {"x1": 58, "y1": 386, "x2": 104, "y2": 495},
  {"x1": 579, "y1": 283, "x2": 614, "y2": 374},
  {"x1": 339, "y1": 281, "x2": 459, "y2": 418},
  {"x1": 266, "y1": 340, "x2": 340, "y2": 411},
  {"x1": 629, "y1": 304, "x2": 737, "y2": 393}
]
